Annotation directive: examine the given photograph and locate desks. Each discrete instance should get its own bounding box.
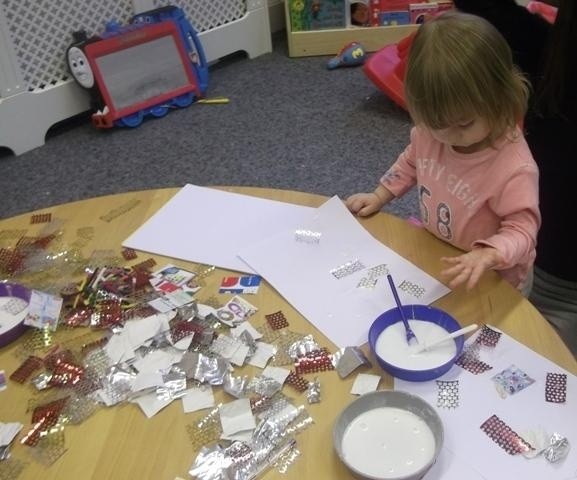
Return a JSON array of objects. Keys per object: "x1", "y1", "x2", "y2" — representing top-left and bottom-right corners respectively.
[{"x1": 0, "y1": 184, "x2": 574, "y2": 480}]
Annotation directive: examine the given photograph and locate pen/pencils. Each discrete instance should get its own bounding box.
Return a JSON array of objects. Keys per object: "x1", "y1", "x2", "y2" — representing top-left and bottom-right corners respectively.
[{"x1": 195, "y1": 96, "x2": 231, "y2": 104}]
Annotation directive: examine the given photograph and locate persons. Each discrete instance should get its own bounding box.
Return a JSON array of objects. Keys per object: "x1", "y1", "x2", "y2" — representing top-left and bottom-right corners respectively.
[
  {"x1": 452, "y1": 0, "x2": 576, "y2": 362},
  {"x1": 344, "y1": 9, "x2": 541, "y2": 302}
]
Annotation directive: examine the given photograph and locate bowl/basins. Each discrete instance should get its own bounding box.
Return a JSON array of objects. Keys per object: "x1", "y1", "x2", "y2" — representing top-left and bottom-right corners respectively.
[
  {"x1": 0, "y1": 284, "x2": 34, "y2": 347},
  {"x1": 334, "y1": 390, "x2": 445, "y2": 479},
  {"x1": 369, "y1": 304, "x2": 464, "y2": 382}
]
